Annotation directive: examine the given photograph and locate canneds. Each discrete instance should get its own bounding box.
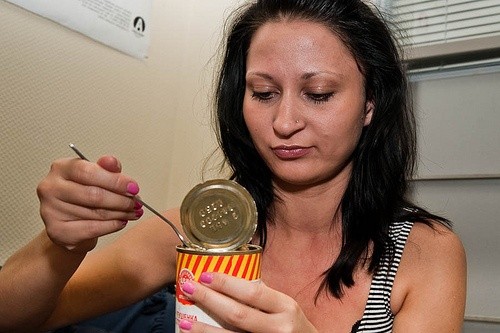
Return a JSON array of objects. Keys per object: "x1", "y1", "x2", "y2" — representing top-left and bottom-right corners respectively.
[{"x1": 175, "y1": 179, "x2": 263, "y2": 333}]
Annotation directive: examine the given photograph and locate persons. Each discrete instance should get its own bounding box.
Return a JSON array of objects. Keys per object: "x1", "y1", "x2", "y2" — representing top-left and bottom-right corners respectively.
[{"x1": 0, "y1": 0, "x2": 468, "y2": 333}]
